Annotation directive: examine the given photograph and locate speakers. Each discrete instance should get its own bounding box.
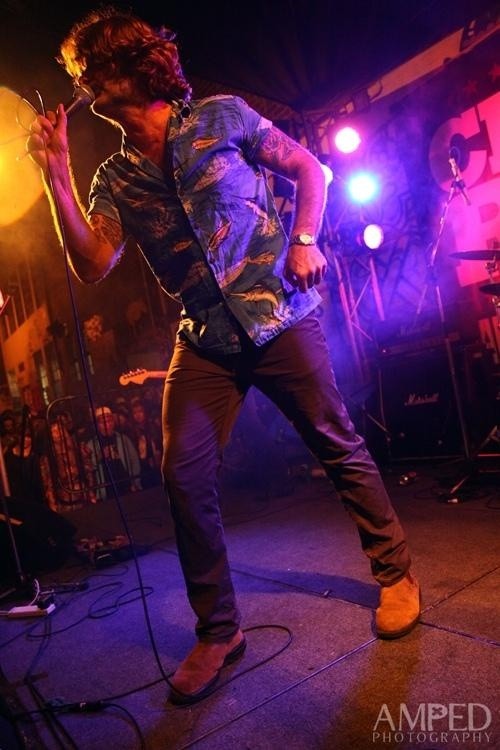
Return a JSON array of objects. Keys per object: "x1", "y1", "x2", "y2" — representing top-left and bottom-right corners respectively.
[{"x1": 377, "y1": 341, "x2": 475, "y2": 459}]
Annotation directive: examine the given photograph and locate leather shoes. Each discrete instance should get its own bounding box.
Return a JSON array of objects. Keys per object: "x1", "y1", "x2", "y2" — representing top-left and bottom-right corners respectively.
[
  {"x1": 374, "y1": 568, "x2": 421, "y2": 639},
  {"x1": 169, "y1": 627, "x2": 248, "y2": 705}
]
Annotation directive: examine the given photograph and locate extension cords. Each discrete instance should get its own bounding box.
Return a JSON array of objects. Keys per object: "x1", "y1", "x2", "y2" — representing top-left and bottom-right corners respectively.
[{"x1": 7, "y1": 604, "x2": 55, "y2": 617}]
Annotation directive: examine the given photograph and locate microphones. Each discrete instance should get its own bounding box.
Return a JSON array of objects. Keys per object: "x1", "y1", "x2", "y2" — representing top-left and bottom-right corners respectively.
[
  {"x1": 64, "y1": 83, "x2": 95, "y2": 117},
  {"x1": 449, "y1": 157, "x2": 471, "y2": 207}
]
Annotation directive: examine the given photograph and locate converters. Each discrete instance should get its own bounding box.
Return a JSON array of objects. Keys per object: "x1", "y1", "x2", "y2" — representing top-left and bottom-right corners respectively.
[{"x1": 35, "y1": 592, "x2": 53, "y2": 608}]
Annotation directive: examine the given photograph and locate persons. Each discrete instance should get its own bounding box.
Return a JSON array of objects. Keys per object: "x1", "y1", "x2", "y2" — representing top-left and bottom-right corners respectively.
[
  {"x1": 0, "y1": 383, "x2": 177, "y2": 510},
  {"x1": 23, "y1": 4, "x2": 426, "y2": 705},
  {"x1": 0, "y1": 412, "x2": 90, "y2": 567}
]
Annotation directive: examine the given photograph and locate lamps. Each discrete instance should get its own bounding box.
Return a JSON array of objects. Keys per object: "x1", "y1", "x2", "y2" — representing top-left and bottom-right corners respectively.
[{"x1": 336, "y1": 222, "x2": 385, "y2": 255}]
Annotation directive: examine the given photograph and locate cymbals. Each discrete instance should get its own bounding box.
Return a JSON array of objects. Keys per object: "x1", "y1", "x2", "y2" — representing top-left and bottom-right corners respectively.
[
  {"x1": 479, "y1": 282, "x2": 500, "y2": 295},
  {"x1": 447, "y1": 249, "x2": 500, "y2": 261}
]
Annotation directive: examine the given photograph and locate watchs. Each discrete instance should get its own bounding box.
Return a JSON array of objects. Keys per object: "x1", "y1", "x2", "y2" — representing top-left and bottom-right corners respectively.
[{"x1": 288, "y1": 232, "x2": 317, "y2": 247}]
